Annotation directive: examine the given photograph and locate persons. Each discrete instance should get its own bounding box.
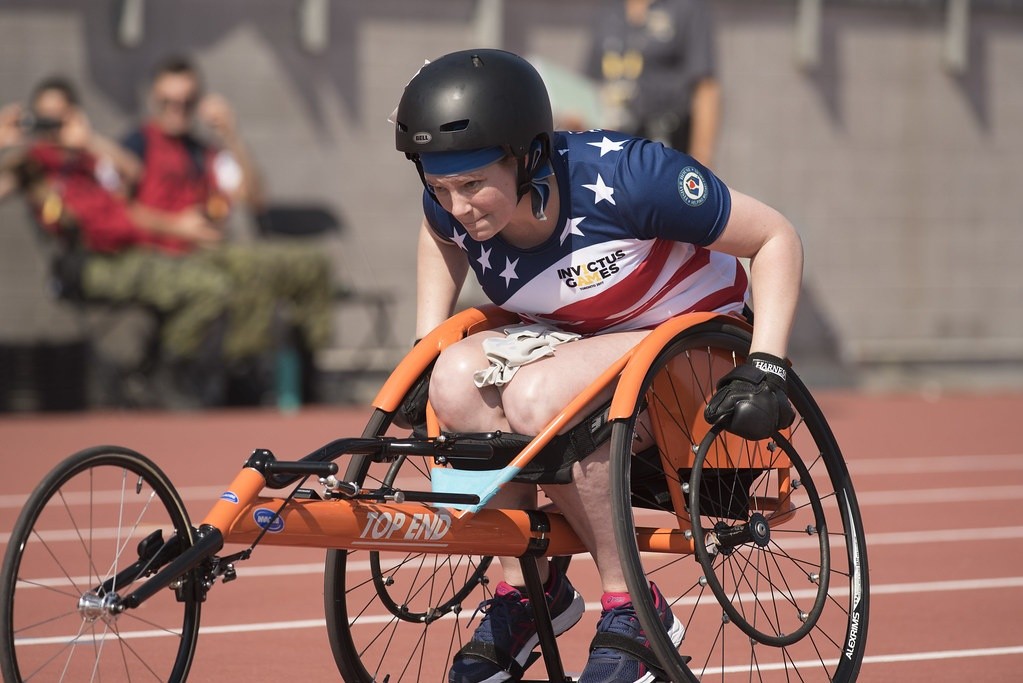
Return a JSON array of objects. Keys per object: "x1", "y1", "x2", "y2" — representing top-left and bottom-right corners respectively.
[
  {"x1": 395, "y1": 47, "x2": 805, "y2": 683},
  {"x1": 582, "y1": 0, "x2": 723, "y2": 171},
  {"x1": 16, "y1": 51, "x2": 331, "y2": 408}
]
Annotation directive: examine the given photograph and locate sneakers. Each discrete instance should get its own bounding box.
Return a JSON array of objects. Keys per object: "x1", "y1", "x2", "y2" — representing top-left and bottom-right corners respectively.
[
  {"x1": 448, "y1": 562, "x2": 585, "y2": 683},
  {"x1": 578, "y1": 580, "x2": 686, "y2": 683}
]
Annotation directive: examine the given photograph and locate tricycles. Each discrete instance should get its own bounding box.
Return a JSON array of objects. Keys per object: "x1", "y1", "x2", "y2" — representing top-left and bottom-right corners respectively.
[{"x1": 0, "y1": 305, "x2": 871, "y2": 683}]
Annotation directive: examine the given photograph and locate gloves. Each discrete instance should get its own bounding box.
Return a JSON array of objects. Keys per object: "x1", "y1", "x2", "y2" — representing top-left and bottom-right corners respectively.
[
  {"x1": 705, "y1": 352, "x2": 796, "y2": 441},
  {"x1": 393, "y1": 339, "x2": 441, "y2": 427}
]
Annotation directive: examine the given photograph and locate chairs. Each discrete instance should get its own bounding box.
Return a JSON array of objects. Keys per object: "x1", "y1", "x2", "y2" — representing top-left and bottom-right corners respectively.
[{"x1": 35, "y1": 203, "x2": 408, "y2": 410}]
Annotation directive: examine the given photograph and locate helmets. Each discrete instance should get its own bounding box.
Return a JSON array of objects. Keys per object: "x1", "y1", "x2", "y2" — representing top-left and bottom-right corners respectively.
[{"x1": 396, "y1": 48, "x2": 554, "y2": 161}]
